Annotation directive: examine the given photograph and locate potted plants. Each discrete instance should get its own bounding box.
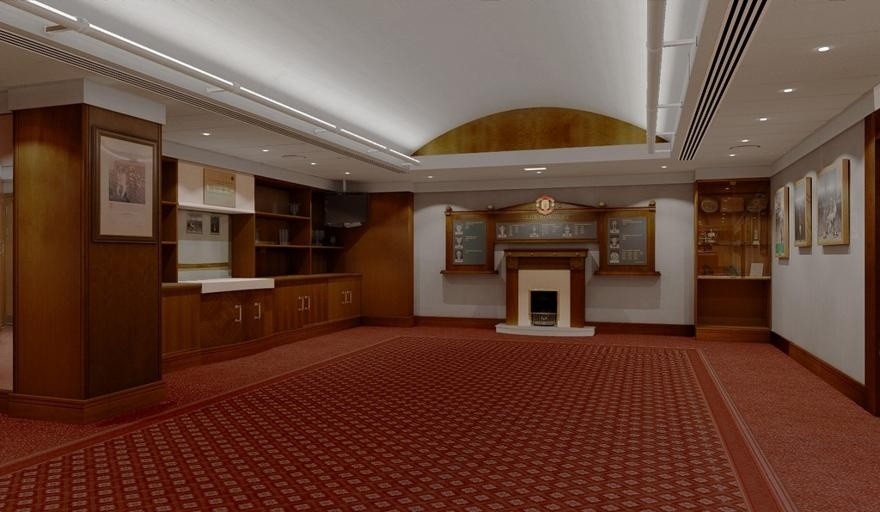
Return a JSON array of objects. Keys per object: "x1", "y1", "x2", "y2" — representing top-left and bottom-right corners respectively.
[
  {"x1": 794, "y1": 177, "x2": 811, "y2": 247},
  {"x1": 775, "y1": 186, "x2": 789, "y2": 259},
  {"x1": 816, "y1": 159, "x2": 850, "y2": 246},
  {"x1": 91, "y1": 123, "x2": 157, "y2": 245}
]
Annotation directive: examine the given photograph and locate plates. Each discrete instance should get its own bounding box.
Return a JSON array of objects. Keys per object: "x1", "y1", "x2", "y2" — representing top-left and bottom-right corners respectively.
[
  {"x1": 440, "y1": 195, "x2": 660, "y2": 276},
  {"x1": 200, "y1": 279, "x2": 273, "y2": 365},
  {"x1": 160, "y1": 155, "x2": 178, "y2": 283},
  {"x1": 693, "y1": 177, "x2": 773, "y2": 344},
  {"x1": 272, "y1": 277, "x2": 328, "y2": 348},
  {"x1": 328, "y1": 275, "x2": 362, "y2": 333},
  {"x1": 231, "y1": 176, "x2": 348, "y2": 277}
]
